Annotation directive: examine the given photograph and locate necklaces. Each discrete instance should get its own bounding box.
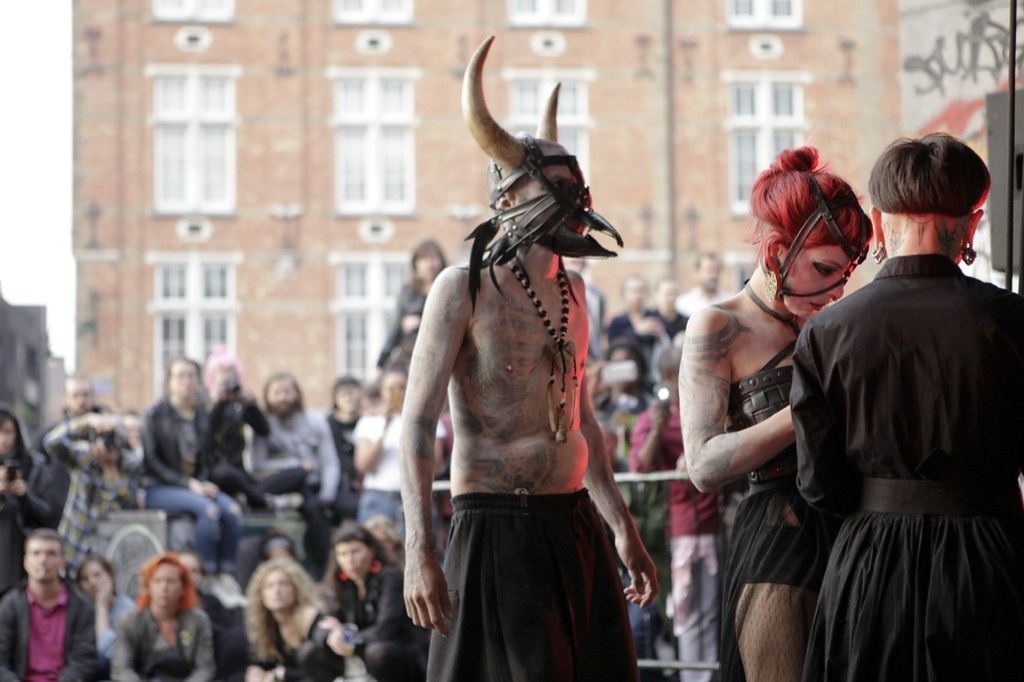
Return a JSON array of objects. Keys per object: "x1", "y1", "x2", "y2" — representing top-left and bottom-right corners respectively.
[{"x1": 506, "y1": 258, "x2": 579, "y2": 443}]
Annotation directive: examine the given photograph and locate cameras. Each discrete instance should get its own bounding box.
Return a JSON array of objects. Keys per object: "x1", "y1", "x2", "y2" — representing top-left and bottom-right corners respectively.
[{"x1": 223, "y1": 380, "x2": 240, "y2": 392}]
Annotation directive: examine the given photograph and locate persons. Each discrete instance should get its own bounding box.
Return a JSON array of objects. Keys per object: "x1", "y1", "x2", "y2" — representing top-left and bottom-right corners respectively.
[
  {"x1": 790, "y1": 132, "x2": 1024, "y2": 682},
  {"x1": 374, "y1": 238, "x2": 450, "y2": 378},
  {"x1": 75, "y1": 555, "x2": 140, "y2": 659},
  {"x1": 109, "y1": 553, "x2": 214, "y2": 682},
  {"x1": 399, "y1": 37, "x2": 658, "y2": 682},
  {"x1": 563, "y1": 249, "x2": 735, "y2": 682},
  {"x1": 0, "y1": 360, "x2": 454, "y2": 682},
  {"x1": 678, "y1": 147, "x2": 873, "y2": 682}
]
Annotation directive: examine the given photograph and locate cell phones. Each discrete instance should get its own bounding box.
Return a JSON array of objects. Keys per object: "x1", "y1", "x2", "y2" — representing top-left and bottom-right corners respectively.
[
  {"x1": 602, "y1": 360, "x2": 638, "y2": 384},
  {"x1": 6, "y1": 463, "x2": 21, "y2": 483}
]
